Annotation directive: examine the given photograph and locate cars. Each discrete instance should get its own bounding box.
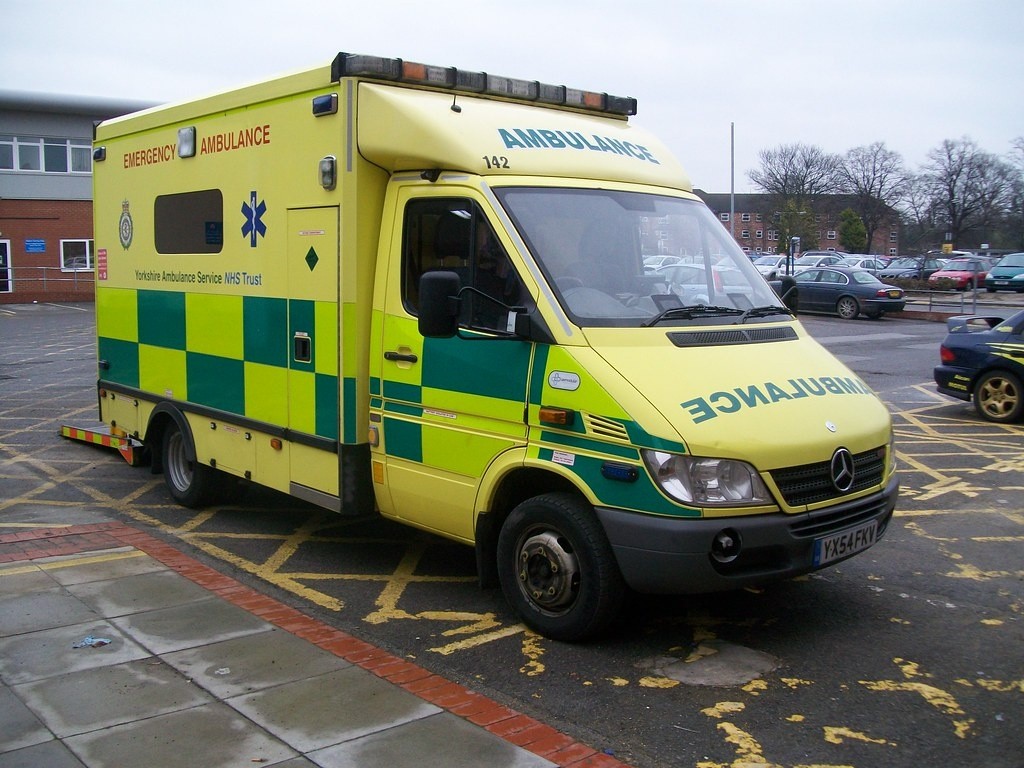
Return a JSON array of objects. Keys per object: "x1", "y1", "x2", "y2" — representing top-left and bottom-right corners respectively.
[
  {"x1": 933, "y1": 308, "x2": 1024, "y2": 423},
  {"x1": 928, "y1": 258, "x2": 993, "y2": 293},
  {"x1": 641, "y1": 250, "x2": 1004, "y2": 305},
  {"x1": 765, "y1": 265, "x2": 906, "y2": 319},
  {"x1": 983, "y1": 253, "x2": 1024, "y2": 293}
]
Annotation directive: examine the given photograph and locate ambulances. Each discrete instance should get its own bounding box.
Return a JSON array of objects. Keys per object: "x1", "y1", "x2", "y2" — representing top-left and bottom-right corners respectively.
[{"x1": 89, "y1": 50, "x2": 899, "y2": 645}]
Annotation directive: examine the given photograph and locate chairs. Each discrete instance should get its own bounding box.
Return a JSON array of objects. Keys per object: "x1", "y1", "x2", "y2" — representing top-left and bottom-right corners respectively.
[
  {"x1": 866, "y1": 262, "x2": 870, "y2": 268},
  {"x1": 823, "y1": 273, "x2": 831, "y2": 281},
  {"x1": 834, "y1": 274, "x2": 843, "y2": 282},
  {"x1": 422, "y1": 213, "x2": 506, "y2": 308}
]
